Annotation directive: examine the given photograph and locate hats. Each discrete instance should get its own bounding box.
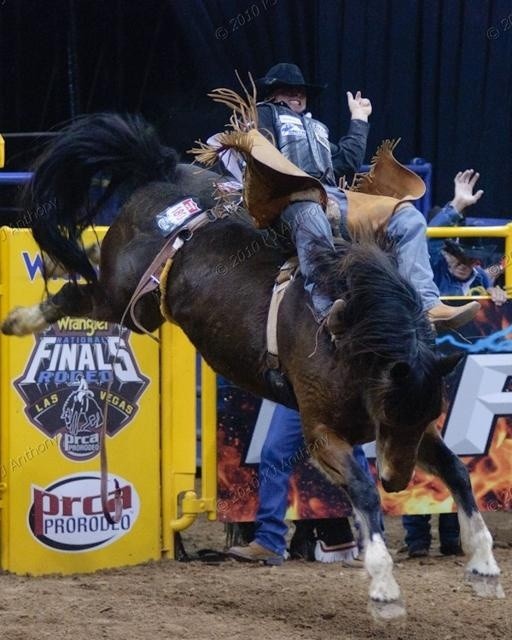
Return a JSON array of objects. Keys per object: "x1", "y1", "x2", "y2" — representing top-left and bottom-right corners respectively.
[{"x1": 248, "y1": 62, "x2": 328, "y2": 92}]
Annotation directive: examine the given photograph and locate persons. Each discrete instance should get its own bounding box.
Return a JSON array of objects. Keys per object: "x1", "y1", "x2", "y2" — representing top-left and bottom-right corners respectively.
[
  {"x1": 207, "y1": 63, "x2": 481, "y2": 342},
  {"x1": 428, "y1": 169, "x2": 511, "y2": 308},
  {"x1": 388, "y1": 510, "x2": 467, "y2": 561},
  {"x1": 226, "y1": 402, "x2": 386, "y2": 563}
]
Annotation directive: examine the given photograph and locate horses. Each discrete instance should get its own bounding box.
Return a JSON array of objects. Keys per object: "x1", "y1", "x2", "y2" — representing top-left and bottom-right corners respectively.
[{"x1": 0, "y1": 106, "x2": 507, "y2": 628}]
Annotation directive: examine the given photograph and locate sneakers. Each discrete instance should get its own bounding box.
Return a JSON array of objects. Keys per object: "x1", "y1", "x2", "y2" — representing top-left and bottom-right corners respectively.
[
  {"x1": 425, "y1": 301, "x2": 481, "y2": 340},
  {"x1": 412, "y1": 547, "x2": 428, "y2": 556},
  {"x1": 342, "y1": 551, "x2": 365, "y2": 568},
  {"x1": 328, "y1": 299, "x2": 347, "y2": 335},
  {"x1": 439, "y1": 542, "x2": 465, "y2": 556},
  {"x1": 227, "y1": 541, "x2": 284, "y2": 566}
]
